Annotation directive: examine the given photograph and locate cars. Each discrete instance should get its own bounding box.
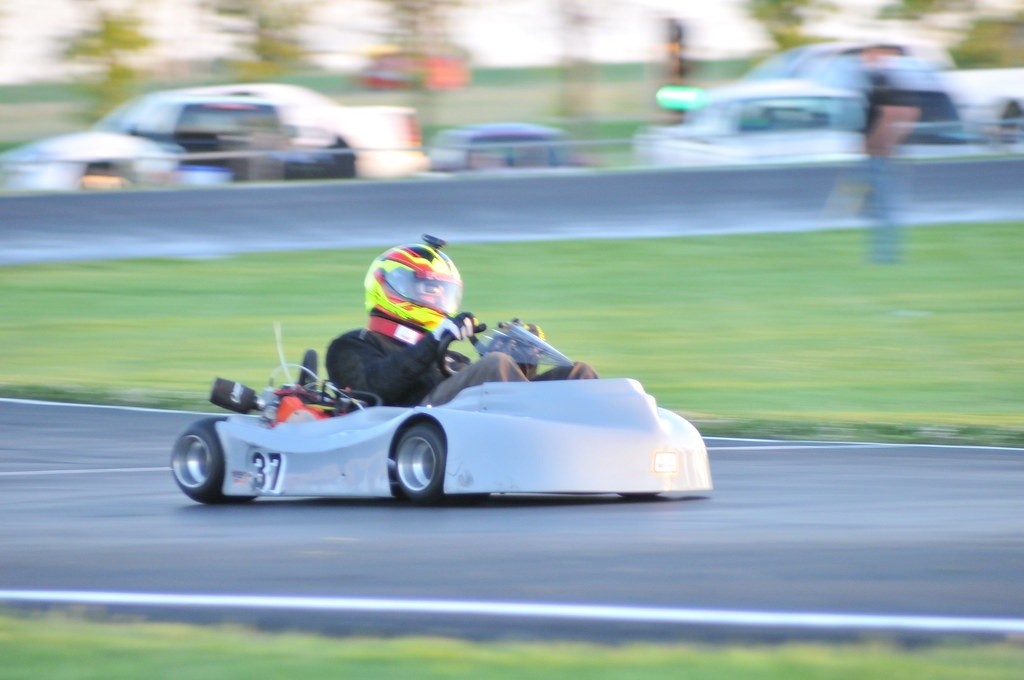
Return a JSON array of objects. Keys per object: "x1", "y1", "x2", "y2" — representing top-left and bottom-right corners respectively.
[
  {"x1": 634, "y1": 38, "x2": 1024, "y2": 151},
  {"x1": 4, "y1": 84, "x2": 588, "y2": 196}
]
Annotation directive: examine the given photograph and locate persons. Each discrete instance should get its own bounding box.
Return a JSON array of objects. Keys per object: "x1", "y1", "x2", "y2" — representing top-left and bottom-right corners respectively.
[
  {"x1": 860, "y1": 42, "x2": 922, "y2": 265},
  {"x1": 326, "y1": 243, "x2": 598, "y2": 409}
]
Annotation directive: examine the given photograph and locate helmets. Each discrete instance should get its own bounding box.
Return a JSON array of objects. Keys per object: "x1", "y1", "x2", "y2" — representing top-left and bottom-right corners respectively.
[{"x1": 364, "y1": 243, "x2": 462, "y2": 345}]
